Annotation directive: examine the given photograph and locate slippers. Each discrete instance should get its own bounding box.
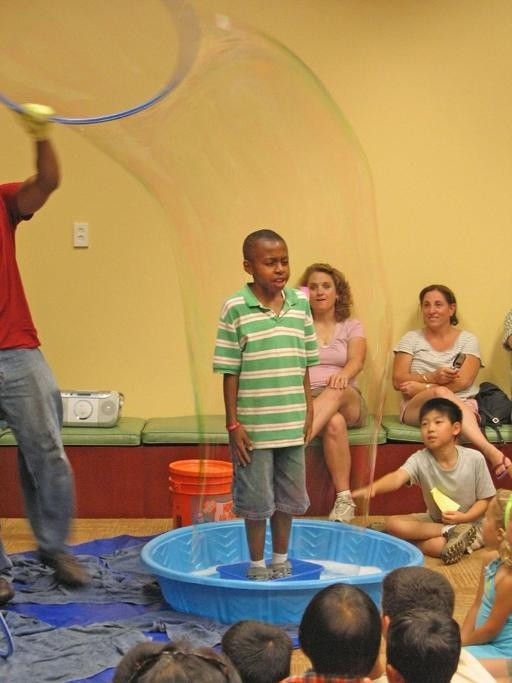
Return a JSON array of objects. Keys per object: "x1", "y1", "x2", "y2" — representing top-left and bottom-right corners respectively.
[{"x1": 491, "y1": 450, "x2": 512, "y2": 485}]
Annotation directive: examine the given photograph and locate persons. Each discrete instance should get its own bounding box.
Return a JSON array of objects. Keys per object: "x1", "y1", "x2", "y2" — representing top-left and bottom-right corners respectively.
[
  {"x1": 381, "y1": 566, "x2": 455, "y2": 641},
  {"x1": 392, "y1": 283, "x2": 512, "y2": 480},
  {"x1": 112, "y1": 641, "x2": 243, "y2": 683},
  {"x1": 277, "y1": 583, "x2": 381, "y2": 683},
  {"x1": 501, "y1": 307, "x2": 512, "y2": 351},
  {"x1": 212, "y1": 230, "x2": 320, "y2": 584},
  {"x1": 222, "y1": 620, "x2": 293, "y2": 683},
  {"x1": 352, "y1": 398, "x2": 496, "y2": 565},
  {"x1": 461, "y1": 489, "x2": 512, "y2": 682},
  {"x1": 292, "y1": 263, "x2": 367, "y2": 523},
  {"x1": 386, "y1": 607, "x2": 460, "y2": 683},
  {"x1": 0, "y1": 101, "x2": 90, "y2": 601}
]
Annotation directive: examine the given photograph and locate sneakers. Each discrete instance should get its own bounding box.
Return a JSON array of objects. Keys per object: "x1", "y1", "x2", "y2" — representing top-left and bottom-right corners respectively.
[
  {"x1": 244, "y1": 566, "x2": 271, "y2": 581},
  {"x1": 38, "y1": 545, "x2": 91, "y2": 589},
  {"x1": 272, "y1": 562, "x2": 293, "y2": 578},
  {"x1": 329, "y1": 495, "x2": 357, "y2": 523},
  {"x1": 440, "y1": 522, "x2": 476, "y2": 566},
  {"x1": 1, "y1": 573, "x2": 21, "y2": 609}
]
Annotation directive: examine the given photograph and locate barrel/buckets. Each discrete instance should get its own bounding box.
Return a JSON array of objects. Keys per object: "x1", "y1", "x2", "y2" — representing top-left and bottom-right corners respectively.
[{"x1": 167, "y1": 460, "x2": 239, "y2": 531}]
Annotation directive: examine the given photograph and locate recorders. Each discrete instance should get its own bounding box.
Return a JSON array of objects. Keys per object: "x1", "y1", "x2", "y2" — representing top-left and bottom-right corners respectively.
[{"x1": 60, "y1": 390, "x2": 123, "y2": 427}]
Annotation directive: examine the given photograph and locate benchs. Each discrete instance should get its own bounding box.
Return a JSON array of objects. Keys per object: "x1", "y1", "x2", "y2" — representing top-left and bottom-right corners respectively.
[{"x1": 0, "y1": 416, "x2": 512, "y2": 517}]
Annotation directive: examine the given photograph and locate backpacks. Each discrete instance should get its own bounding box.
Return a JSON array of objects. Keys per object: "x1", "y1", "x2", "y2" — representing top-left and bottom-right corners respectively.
[{"x1": 468, "y1": 381, "x2": 511, "y2": 433}]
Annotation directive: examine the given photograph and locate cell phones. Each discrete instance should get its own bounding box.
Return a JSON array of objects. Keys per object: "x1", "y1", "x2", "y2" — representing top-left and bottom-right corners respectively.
[{"x1": 450, "y1": 351, "x2": 467, "y2": 370}]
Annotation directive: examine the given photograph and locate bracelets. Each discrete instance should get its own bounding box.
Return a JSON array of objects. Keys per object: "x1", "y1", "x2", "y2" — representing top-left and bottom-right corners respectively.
[
  {"x1": 225, "y1": 421, "x2": 241, "y2": 430},
  {"x1": 426, "y1": 384, "x2": 431, "y2": 388},
  {"x1": 421, "y1": 373, "x2": 429, "y2": 382}
]
